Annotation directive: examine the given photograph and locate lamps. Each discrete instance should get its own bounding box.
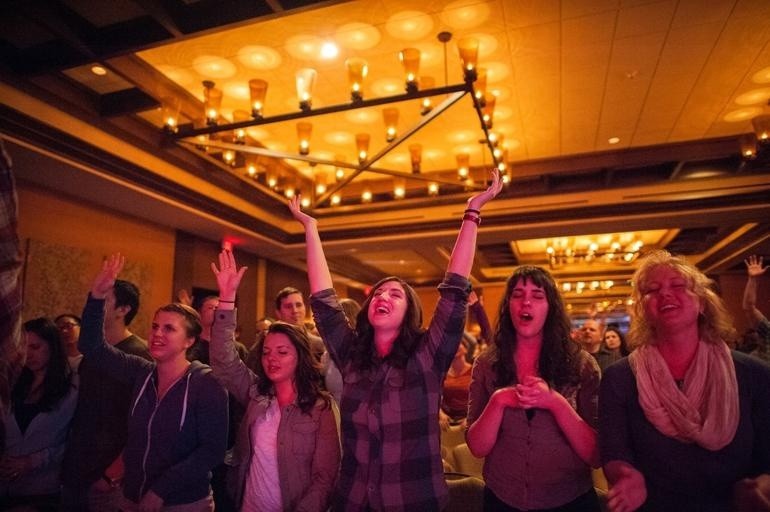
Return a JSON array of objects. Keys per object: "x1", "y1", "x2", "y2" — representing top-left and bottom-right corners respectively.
[
  {"x1": 545, "y1": 241, "x2": 642, "y2": 294},
  {"x1": 157, "y1": 37, "x2": 512, "y2": 216}
]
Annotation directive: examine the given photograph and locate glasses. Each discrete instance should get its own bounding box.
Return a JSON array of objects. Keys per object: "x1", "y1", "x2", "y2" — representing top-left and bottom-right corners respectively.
[{"x1": 56, "y1": 322, "x2": 81, "y2": 331}]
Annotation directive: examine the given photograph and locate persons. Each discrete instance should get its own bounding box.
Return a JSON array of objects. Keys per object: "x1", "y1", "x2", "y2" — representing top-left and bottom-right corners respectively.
[{"x1": 2, "y1": 146, "x2": 769, "y2": 512}]
